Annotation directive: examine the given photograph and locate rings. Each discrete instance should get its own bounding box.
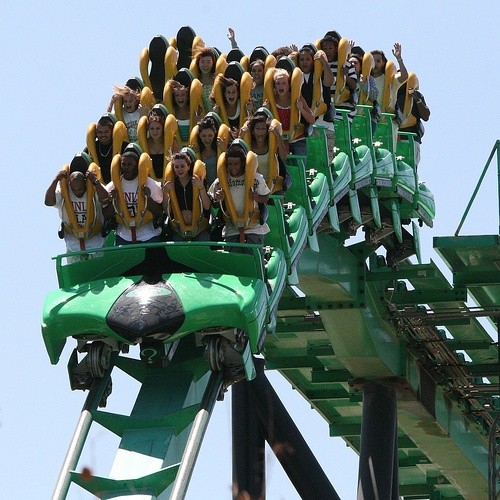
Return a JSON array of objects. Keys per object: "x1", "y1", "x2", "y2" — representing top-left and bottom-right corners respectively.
[{"x1": 216, "y1": 192, "x2": 220, "y2": 195}]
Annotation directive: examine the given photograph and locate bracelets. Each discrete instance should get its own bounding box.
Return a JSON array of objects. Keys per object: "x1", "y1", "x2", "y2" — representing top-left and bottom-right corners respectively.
[
  {"x1": 231, "y1": 42, "x2": 237, "y2": 45},
  {"x1": 416, "y1": 98, "x2": 422, "y2": 104},
  {"x1": 198, "y1": 185, "x2": 205, "y2": 192},
  {"x1": 397, "y1": 58, "x2": 404, "y2": 65}
]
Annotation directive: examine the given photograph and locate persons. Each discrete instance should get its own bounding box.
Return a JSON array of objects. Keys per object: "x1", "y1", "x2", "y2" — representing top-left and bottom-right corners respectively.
[
  {"x1": 102, "y1": 151, "x2": 164, "y2": 249},
  {"x1": 75, "y1": 24, "x2": 430, "y2": 180},
  {"x1": 207, "y1": 145, "x2": 272, "y2": 255},
  {"x1": 44, "y1": 169, "x2": 110, "y2": 266},
  {"x1": 163, "y1": 152, "x2": 212, "y2": 244}
]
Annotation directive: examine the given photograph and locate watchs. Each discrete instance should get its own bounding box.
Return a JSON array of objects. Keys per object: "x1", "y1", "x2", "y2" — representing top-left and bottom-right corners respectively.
[{"x1": 92, "y1": 179, "x2": 99, "y2": 185}]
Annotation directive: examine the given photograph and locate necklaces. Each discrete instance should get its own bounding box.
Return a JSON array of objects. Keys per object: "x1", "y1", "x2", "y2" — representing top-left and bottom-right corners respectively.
[
  {"x1": 98, "y1": 141, "x2": 113, "y2": 158},
  {"x1": 150, "y1": 141, "x2": 163, "y2": 154}
]
